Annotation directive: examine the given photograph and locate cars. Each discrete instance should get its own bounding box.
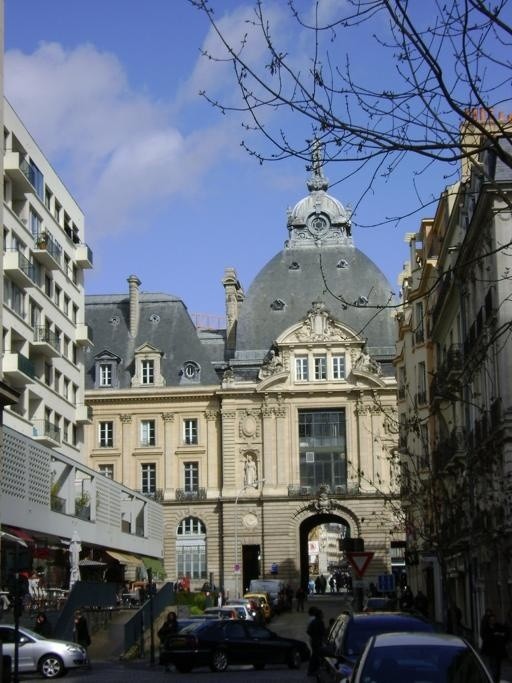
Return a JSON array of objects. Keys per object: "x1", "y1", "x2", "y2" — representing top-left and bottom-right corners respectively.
[
  {"x1": 317, "y1": 612, "x2": 439, "y2": 683},
  {"x1": 169, "y1": 618, "x2": 312, "y2": 671},
  {"x1": 341, "y1": 632, "x2": 496, "y2": 683},
  {"x1": 173, "y1": 598, "x2": 265, "y2": 630},
  {"x1": 362, "y1": 597, "x2": 389, "y2": 613},
  {"x1": 0, "y1": 625, "x2": 87, "y2": 678}
]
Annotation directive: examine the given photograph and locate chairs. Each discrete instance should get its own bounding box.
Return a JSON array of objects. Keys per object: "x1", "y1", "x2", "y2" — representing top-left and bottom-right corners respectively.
[
  {"x1": 438, "y1": 653, "x2": 456, "y2": 683},
  {"x1": 376, "y1": 656, "x2": 405, "y2": 683}
]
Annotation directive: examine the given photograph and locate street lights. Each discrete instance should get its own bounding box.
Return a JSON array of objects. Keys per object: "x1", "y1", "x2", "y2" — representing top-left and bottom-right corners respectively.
[
  {"x1": 68, "y1": 530, "x2": 82, "y2": 586},
  {"x1": 235, "y1": 485, "x2": 264, "y2": 601}
]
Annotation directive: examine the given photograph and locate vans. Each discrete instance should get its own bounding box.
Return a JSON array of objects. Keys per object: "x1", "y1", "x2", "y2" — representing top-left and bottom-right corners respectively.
[{"x1": 249, "y1": 579, "x2": 288, "y2": 606}]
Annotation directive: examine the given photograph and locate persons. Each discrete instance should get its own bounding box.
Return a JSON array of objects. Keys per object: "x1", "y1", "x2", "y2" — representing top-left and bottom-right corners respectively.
[
  {"x1": 156, "y1": 611, "x2": 181, "y2": 672},
  {"x1": 281, "y1": 581, "x2": 294, "y2": 614},
  {"x1": 322, "y1": 616, "x2": 334, "y2": 636},
  {"x1": 352, "y1": 579, "x2": 428, "y2": 614},
  {"x1": 73, "y1": 609, "x2": 94, "y2": 672},
  {"x1": 295, "y1": 583, "x2": 309, "y2": 612},
  {"x1": 445, "y1": 604, "x2": 473, "y2": 642},
  {"x1": 313, "y1": 572, "x2": 352, "y2": 593},
  {"x1": 34, "y1": 612, "x2": 51, "y2": 637},
  {"x1": 476, "y1": 598, "x2": 505, "y2": 682},
  {"x1": 305, "y1": 604, "x2": 326, "y2": 674}
]
